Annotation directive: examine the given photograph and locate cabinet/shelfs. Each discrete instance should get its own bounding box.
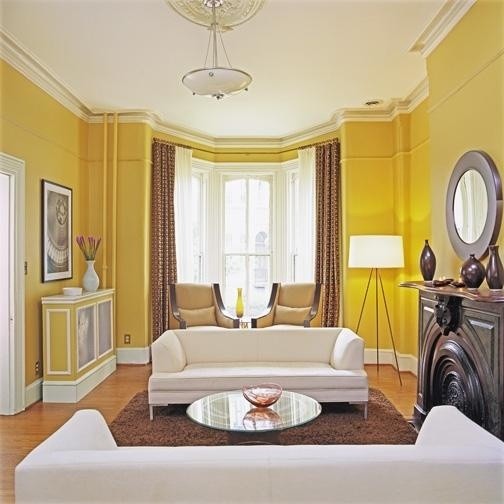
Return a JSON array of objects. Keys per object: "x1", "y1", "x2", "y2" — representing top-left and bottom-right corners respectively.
[
  {"x1": 398, "y1": 281, "x2": 504, "y2": 440},
  {"x1": 41, "y1": 288, "x2": 117, "y2": 403}
]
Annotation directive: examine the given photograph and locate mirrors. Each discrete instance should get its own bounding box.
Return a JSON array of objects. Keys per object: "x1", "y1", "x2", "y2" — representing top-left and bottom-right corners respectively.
[{"x1": 446, "y1": 150, "x2": 502, "y2": 261}]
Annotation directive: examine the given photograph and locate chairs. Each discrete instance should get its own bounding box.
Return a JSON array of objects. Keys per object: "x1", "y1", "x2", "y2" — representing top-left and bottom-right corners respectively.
[
  {"x1": 168, "y1": 283, "x2": 240, "y2": 329},
  {"x1": 251, "y1": 283, "x2": 320, "y2": 328}
]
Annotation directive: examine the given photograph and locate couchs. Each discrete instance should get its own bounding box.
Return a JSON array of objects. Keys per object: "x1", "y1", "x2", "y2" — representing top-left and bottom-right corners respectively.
[
  {"x1": 148, "y1": 326, "x2": 369, "y2": 421},
  {"x1": 15, "y1": 405, "x2": 504, "y2": 503}
]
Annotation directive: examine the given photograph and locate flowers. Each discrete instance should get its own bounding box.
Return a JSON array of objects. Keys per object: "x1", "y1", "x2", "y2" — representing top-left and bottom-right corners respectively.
[{"x1": 76, "y1": 236, "x2": 101, "y2": 261}]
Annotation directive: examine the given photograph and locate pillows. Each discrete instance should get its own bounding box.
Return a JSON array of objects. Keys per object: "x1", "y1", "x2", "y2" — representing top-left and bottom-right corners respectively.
[
  {"x1": 274, "y1": 303, "x2": 313, "y2": 325},
  {"x1": 178, "y1": 306, "x2": 217, "y2": 326}
]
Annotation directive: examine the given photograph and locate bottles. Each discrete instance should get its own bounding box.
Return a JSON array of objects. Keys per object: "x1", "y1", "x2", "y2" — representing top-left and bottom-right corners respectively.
[{"x1": 235, "y1": 287, "x2": 244, "y2": 318}]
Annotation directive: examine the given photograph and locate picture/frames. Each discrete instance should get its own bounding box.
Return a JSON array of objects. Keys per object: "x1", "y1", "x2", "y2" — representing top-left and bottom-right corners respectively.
[{"x1": 42, "y1": 179, "x2": 73, "y2": 282}]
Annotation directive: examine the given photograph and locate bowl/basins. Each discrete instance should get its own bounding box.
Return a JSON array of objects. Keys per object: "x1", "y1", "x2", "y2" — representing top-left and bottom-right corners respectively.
[
  {"x1": 63, "y1": 287, "x2": 83, "y2": 296},
  {"x1": 242, "y1": 407, "x2": 282, "y2": 430},
  {"x1": 242, "y1": 383, "x2": 282, "y2": 408}
]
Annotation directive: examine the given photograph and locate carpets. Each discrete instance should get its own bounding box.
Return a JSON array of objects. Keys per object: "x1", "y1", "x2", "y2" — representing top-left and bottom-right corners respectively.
[{"x1": 110, "y1": 390, "x2": 417, "y2": 444}]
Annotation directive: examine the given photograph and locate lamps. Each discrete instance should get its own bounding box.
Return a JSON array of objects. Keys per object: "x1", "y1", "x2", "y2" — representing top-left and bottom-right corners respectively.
[
  {"x1": 348, "y1": 234, "x2": 406, "y2": 387},
  {"x1": 181, "y1": 0, "x2": 251, "y2": 100}
]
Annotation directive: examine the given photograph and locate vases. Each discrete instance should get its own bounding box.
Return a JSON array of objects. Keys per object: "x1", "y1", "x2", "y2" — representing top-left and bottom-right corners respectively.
[
  {"x1": 81, "y1": 261, "x2": 100, "y2": 291},
  {"x1": 236, "y1": 288, "x2": 244, "y2": 318}
]
